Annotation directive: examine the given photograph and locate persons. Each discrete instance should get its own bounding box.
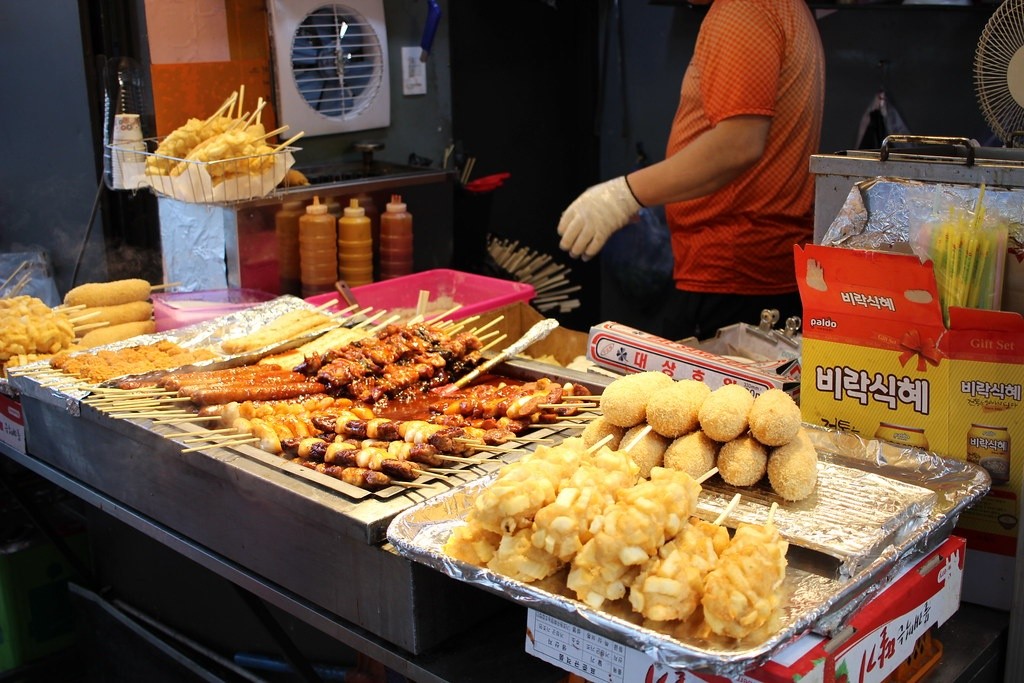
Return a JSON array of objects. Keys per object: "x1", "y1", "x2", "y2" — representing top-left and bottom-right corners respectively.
[{"x1": 557, "y1": 1, "x2": 829, "y2": 338}]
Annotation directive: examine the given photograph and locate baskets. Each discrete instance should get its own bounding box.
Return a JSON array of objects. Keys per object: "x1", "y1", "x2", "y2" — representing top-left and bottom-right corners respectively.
[{"x1": 106, "y1": 136, "x2": 303, "y2": 208}]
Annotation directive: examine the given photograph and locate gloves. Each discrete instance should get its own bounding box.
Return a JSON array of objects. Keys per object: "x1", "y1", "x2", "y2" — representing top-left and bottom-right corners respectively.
[{"x1": 558, "y1": 175, "x2": 645, "y2": 262}]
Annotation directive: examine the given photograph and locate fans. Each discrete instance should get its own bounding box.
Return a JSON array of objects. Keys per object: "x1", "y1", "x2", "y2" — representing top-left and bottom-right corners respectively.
[{"x1": 972, "y1": 0, "x2": 1024, "y2": 147}]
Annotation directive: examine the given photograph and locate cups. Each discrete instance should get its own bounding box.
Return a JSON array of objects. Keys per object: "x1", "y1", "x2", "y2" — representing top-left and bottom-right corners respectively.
[{"x1": 111, "y1": 115, "x2": 145, "y2": 189}]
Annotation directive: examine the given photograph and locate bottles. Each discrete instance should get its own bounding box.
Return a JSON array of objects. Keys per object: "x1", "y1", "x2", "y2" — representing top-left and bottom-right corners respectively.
[
  {"x1": 379, "y1": 196, "x2": 413, "y2": 280},
  {"x1": 337, "y1": 199, "x2": 372, "y2": 285},
  {"x1": 301, "y1": 195, "x2": 337, "y2": 285}
]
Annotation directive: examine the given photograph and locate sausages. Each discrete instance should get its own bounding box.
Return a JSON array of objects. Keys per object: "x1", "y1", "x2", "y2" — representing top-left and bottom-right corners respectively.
[{"x1": 158, "y1": 363, "x2": 327, "y2": 419}]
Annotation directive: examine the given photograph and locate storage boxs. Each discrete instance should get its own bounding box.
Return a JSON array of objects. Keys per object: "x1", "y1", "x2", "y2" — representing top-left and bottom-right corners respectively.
[
  {"x1": 0, "y1": 392, "x2": 26, "y2": 454},
  {"x1": 150, "y1": 288, "x2": 277, "y2": 332},
  {"x1": 305, "y1": 269, "x2": 537, "y2": 326},
  {"x1": 793, "y1": 242, "x2": 1024, "y2": 614},
  {"x1": 524, "y1": 537, "x2": 967, "y2": 683}
]
